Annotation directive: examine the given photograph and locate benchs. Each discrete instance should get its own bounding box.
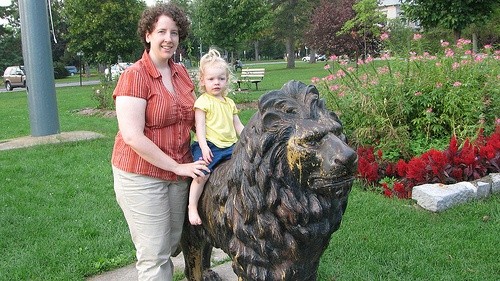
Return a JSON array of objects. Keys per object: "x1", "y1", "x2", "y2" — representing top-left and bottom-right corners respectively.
[{"x1": 237, "y1": 68, "x2": 265, "y2": 90}]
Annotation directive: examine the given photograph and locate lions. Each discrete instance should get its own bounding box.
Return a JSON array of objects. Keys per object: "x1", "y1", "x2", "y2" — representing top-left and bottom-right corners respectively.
[{"x1": 170, "y1": 80, "x2": 360, "y2": 281}]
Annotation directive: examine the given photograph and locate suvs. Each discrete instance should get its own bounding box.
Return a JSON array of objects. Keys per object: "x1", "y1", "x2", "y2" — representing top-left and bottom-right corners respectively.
[{"x1": 1, "y1": 65, "x2": 26, "y2": 91}]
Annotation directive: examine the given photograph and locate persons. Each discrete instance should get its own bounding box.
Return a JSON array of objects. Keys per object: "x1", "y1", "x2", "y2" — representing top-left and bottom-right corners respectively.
[
  {"x1": 188, "y1": 49, "x2": 245, "y2": 225},
  {"x1": 111, "y1": 4, "x2": 210, "y2": 281}
]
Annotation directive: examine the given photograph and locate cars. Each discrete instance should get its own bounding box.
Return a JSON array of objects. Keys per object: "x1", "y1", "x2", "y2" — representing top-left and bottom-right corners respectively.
[
  {"x1": 64, "y1": 66, "x2": 78, "y2": 76},
  {"x1": 104, "y1": 62, "x2": 134, "y2": 82},
  {"x1": 301, "y1": 53, "x2": 326, "y2": 62}
]
